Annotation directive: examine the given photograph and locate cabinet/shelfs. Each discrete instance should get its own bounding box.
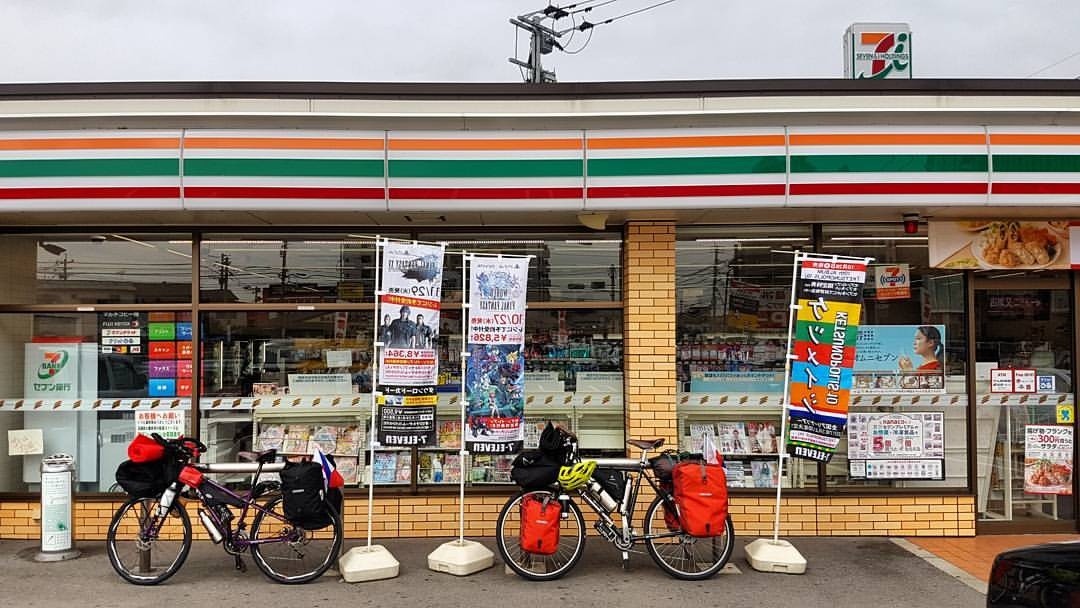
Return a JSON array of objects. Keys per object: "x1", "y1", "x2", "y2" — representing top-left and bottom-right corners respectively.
[
  {"x1": 249, "y1": 409, "x2": 629, "y2": 495},
  {"x1": 991, "y1": 397, "x2": 1057, "y2": 523},
  {"x1": 677, "y1": 405, "x2": 899, "y2": 487}
]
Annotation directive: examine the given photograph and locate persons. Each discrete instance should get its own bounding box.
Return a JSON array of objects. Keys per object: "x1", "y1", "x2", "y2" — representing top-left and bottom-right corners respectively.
[
  {"x1": 899, "y1": 327, "x2": 942, "y2": 370},
  {"x1": 383, "y1": 305, "x2": 437, "y2": 349}
]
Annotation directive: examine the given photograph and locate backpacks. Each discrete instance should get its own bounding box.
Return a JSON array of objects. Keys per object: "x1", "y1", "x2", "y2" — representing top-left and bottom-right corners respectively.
[{"x1": 278, "y1": 461, "x2": 342, "y2": 531}]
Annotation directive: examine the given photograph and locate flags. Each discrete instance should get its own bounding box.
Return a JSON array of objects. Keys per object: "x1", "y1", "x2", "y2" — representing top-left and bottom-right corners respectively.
[
  {"x1": 378, "y1": 238, "x2": 438, "y2": 447},
  {"x1": 787, "y1": 255, "x2": 867, "y2": 463},
  {"x1": 464, "y1": 254, "x2": 529, "y2": 455}
]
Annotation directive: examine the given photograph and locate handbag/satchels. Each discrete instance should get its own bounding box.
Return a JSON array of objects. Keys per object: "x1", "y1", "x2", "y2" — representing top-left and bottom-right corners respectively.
[{"x1": 511, "y1": 418, "x2": 578, "y2": 491}]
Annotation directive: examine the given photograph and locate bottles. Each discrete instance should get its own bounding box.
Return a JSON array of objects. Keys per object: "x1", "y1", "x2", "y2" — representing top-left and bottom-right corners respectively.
[
  {"x1": 558, "y1": 492, "x2": 569, "y2": 517},
  {"x1": 155, "y1": 482, "x2": 177, "y2": 516}
]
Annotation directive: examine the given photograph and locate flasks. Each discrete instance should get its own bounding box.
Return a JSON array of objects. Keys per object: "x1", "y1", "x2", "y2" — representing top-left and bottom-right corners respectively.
[
  {"x1": 587, "y1": 476, "x2": 617, "y2": 511},
  {"x1": 198, "y1": 508, "x2": 222, "y2": 544}
]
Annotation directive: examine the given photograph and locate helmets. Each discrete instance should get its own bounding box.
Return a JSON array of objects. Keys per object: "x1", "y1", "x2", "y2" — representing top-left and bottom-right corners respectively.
[{"x1": 557, "y1": 461, "x2": 596, "y2": 491}]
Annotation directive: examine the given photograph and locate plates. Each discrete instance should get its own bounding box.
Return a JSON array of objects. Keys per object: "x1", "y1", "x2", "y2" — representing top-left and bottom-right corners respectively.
[{"x1": 1027, "y1": 464, "x2": 1072, "y2": 491}]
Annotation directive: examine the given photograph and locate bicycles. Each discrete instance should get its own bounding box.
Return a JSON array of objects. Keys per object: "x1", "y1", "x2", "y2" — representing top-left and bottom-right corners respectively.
[
  {"x1": 105, "y1": 434, "x2": 347, "y2": 588},
  {"x1": 496, "y1": 425, "x2": 734, "y2": 582}
]
